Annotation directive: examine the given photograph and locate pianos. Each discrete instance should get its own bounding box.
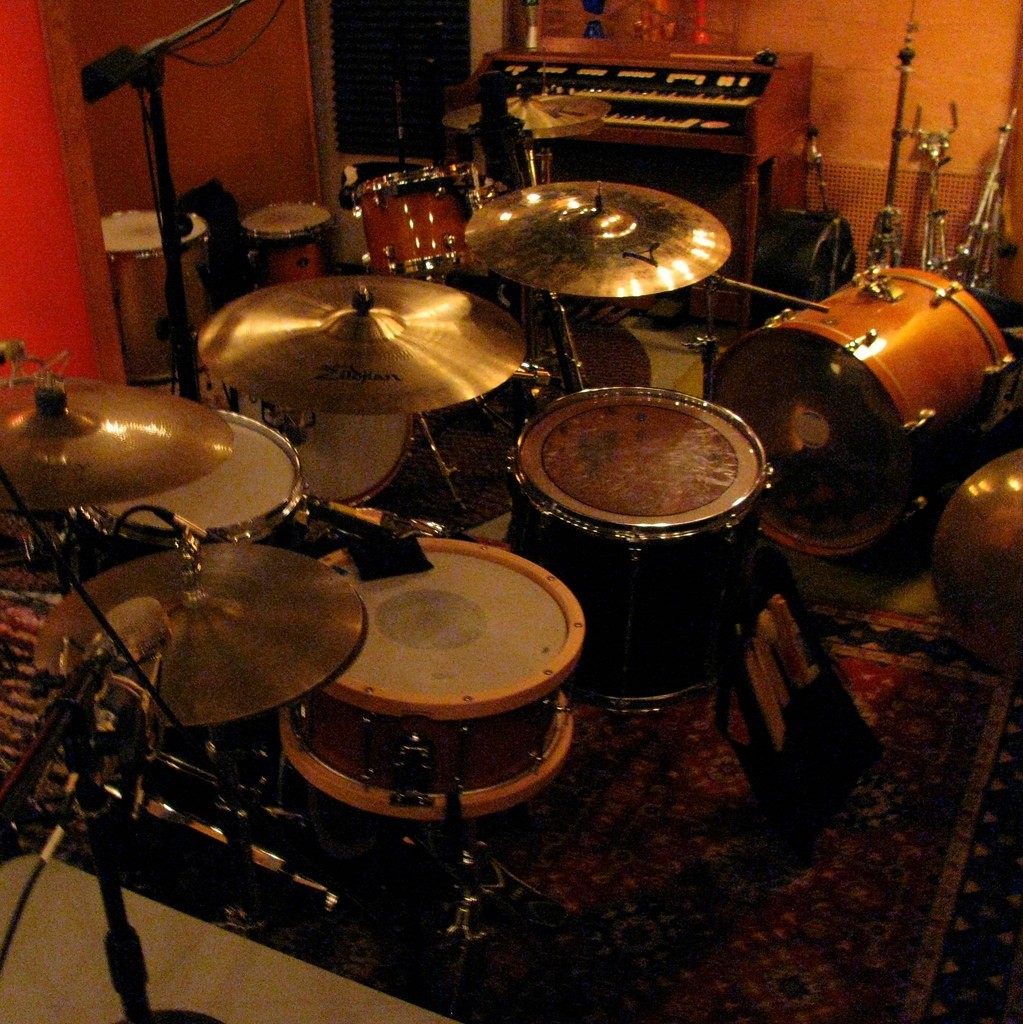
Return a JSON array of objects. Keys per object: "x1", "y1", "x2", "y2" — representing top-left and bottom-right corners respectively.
[{"x1": 447, "y1": 43, "x2": 814, "y2": 303}]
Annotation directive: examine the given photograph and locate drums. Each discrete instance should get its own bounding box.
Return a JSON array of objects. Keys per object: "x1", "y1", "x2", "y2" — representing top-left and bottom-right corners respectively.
[
  {"x1": 198, "y1": 366, "x2": 412, "y2": 504},
  {"x1": 752, "y1": 206, "x2": 856, "y2": 324},
  {"x1": 67, "y1": 405, "x2": 302, "y2": 570},
  {"x1": 956, "y1": 278, "x2": 1023, "y2": 352},
  {"x1": 98, "y1": 205, "x2": 211, "y2": 384},
  {"x1": 351, "y1": 156, "x2": 475, "y2": 286},
  {"x1": 714, "y1": 264, "x2": 1012, "y2": 562},
  {"x1": 279, "y1": 535, "x2": 590, "y2": 818},
  {"x1": 244, "y1": 201, "x2": 330, "y2": 290},
  {"x1": 502, "y1": 386, "x2": 770, "y2": 710}
]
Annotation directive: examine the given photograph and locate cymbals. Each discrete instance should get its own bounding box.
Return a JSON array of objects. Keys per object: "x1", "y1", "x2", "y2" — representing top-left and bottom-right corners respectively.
[
  {"x1": 0, "y1": 370, "x2": 233, "y2": 509},
  {"x1": 464, "y1": 180, "x2": 730, "y2": 300},
  {"x1": 930, "y1": 447, "x2": 1023, "y2": 698},
  {"x1": 433, "y1": 90, "x2": 605, "y2": 142},
  {"x1": 28, "y1": 542, "x2": 366, "y2": 728},
  {"x1": 194, "y1": 268, "x2": 527, "y2": 415}
]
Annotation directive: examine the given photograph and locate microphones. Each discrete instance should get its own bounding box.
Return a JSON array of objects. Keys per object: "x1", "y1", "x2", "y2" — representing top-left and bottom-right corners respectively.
[
  {"x1": 152, "y1": 505, "x2": 225, "y2": 544},
  {"x1": 86, "y1": 597, "x2": 172, "y2": 675}
]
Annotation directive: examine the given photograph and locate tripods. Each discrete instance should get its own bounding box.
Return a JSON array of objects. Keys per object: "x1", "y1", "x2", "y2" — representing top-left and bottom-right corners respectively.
[{"x1": 866, "y1": 34, "x2": 1017, "y2": 293}]
